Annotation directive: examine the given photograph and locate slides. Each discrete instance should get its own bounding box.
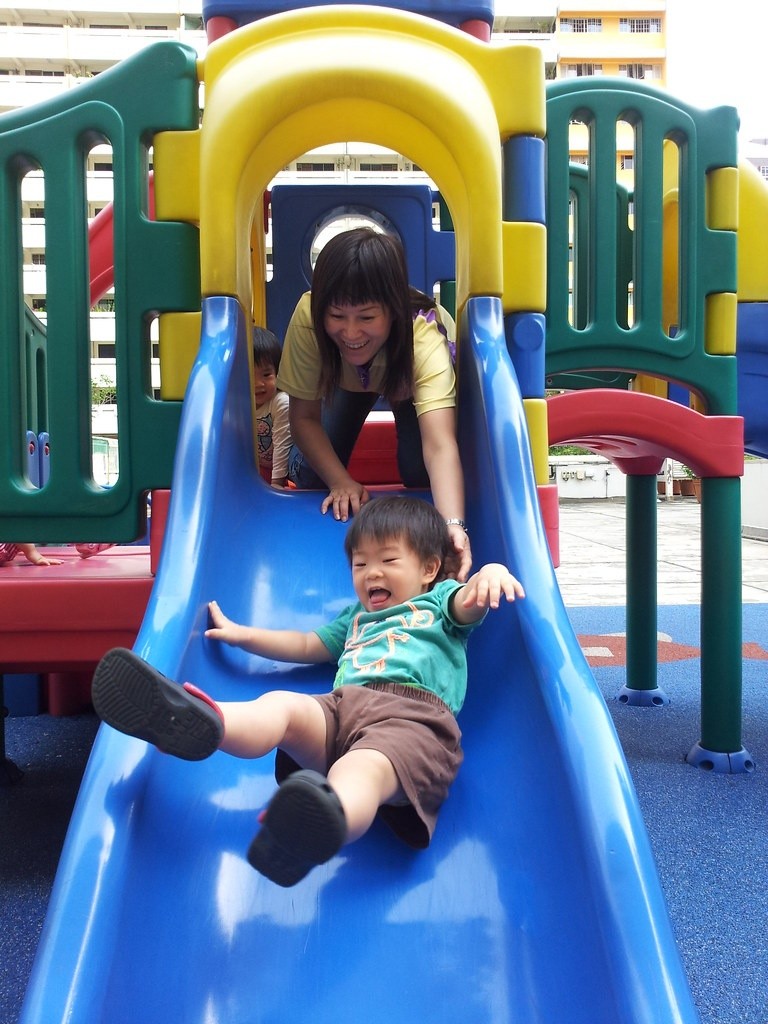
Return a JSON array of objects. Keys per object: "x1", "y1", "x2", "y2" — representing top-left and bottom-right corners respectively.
[{"x1": 17, "y1": 292, "x2": 706, "y2": 1024}]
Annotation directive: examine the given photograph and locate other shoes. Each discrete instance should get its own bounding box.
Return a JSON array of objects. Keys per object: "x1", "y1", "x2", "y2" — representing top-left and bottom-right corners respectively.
[
  {"x1": 92, "y1": 648, "x2": 224, "y2": 762},
  {"x1": 246, "y1": 770, "x2": 347, "y2": 888},
  {"x1": 75, "y1": 543, "x2": 117, "y2": 559}
]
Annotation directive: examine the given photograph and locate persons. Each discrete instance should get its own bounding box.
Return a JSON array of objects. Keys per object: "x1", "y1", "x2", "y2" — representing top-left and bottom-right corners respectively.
[
  {"x1": 92, "y1": 497, "x2": 525, "y2": 887},
  {"x1": 275, "y1": 227, "x2": 474, "y2": 582},
  {"x1": 0, "y1": 543, "x2": 64, "y2": 567},
  {"x1": 253, "y1": 325, "x2": 293, "y2": 489}
]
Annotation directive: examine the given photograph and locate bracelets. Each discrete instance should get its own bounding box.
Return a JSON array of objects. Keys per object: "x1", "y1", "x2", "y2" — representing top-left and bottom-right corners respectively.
[{"x1": 445, "y1": 518, "x2": 468, "y2": 533}]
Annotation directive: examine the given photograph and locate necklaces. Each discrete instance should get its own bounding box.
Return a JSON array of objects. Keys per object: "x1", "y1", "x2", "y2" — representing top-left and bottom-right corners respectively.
[{"x1": 356, "y1": 366, "x2": 369, "y2": 389}]
